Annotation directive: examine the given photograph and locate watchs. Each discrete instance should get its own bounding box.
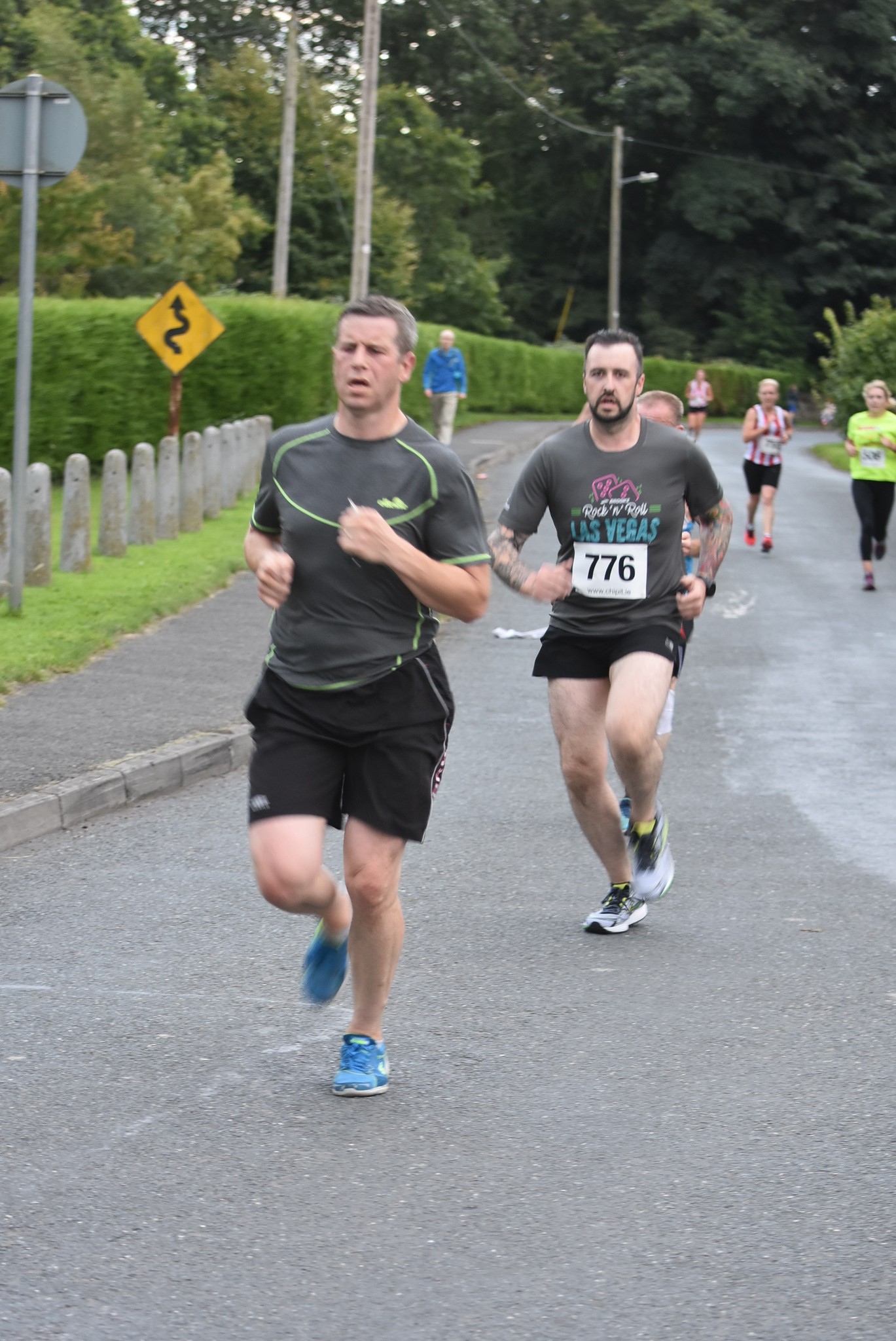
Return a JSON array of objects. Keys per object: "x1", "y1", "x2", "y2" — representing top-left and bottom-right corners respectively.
[{"x1": 695, "y1": 571, "x2": 716, "y2": 597}]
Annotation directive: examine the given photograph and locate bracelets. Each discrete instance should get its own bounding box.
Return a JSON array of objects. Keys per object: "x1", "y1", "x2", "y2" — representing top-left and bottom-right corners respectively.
[{"x1": 786, "y1": 433, "x2": 793, "y2": 441}]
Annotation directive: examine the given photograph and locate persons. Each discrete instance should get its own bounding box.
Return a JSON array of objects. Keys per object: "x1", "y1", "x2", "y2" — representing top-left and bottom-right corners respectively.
[
  {"x1": 742, "y1": 378, "x2": 799, "y2": 553},
  {"x1": 788, "y1": 384, "x2": 837, "y2": 426},
  {"x1": 422, "y1": 329, "x2": 467, "y2": 447},
  {"x1": 843, "y1": 380, "x2": 896, "y2": 592},
  {"x1": 684, "y1": 370, "x2": 714, "y2": 444},
  {"x1": 488, "y1": 327, "x2": 732, "y2": 935},
  {"x1": 245, "y1": 293, "x2": 491, "y2": 1096}
]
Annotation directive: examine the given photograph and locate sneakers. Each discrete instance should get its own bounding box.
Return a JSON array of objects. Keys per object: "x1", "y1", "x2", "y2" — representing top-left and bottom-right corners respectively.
[
  {"x1": 746, "y1": 524, "x2": 756, "y2": 546},
  {"x1": 304, "y1": 917, "x2": 350, "y2": 1000},
  {"x1": 620, "y1": 797, "x2": 633, "y2": 833},
  {"x1": 862, "y1": 571, "x2": 876, "y2": 591},
  {"x1": 623, "y1": 801, "x2": 674, "y2": 901},
  {"x1": 332, "y1": 1034, "x2": 390, "y2": 1096},
  {"x1": 762, "y1": 536, "x2": 772, "y2": 553},
  {"x1": 583, "y1": 883, "x2": 648, "y2": 933},
  {"x1": 875, "y1": 538, "x2": 886, "y2": 558}
]
